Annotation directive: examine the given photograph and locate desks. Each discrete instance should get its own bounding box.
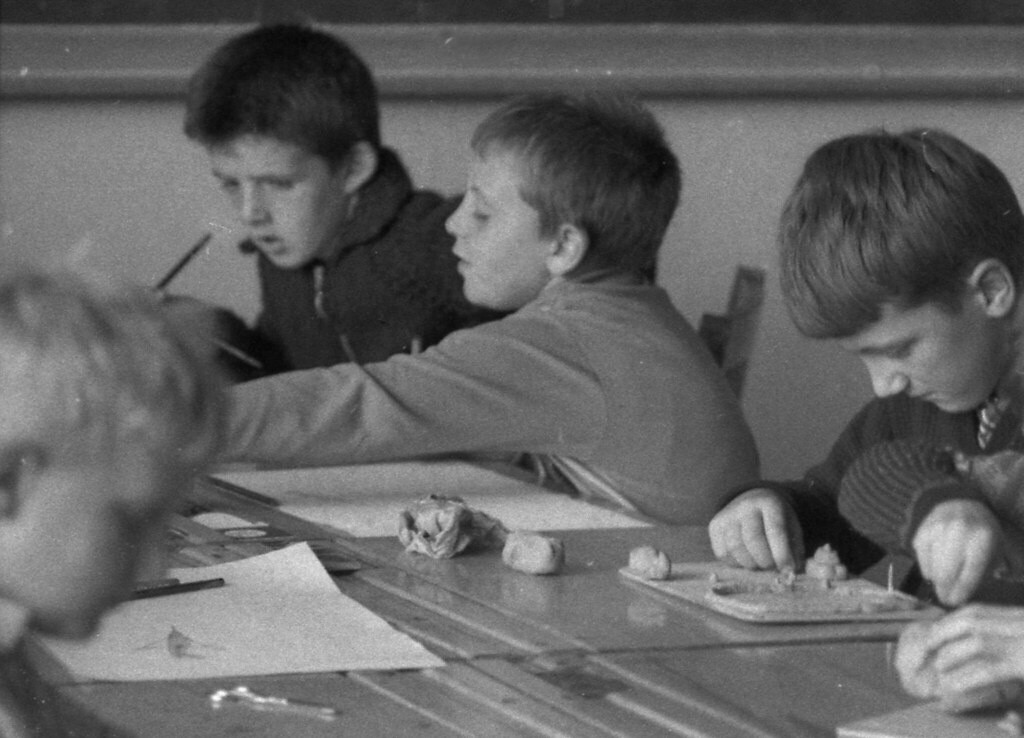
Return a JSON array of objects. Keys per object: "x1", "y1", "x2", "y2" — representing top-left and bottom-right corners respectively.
[{"x1": 23, "y1": 449, "x2": 1024, "y2": 738}]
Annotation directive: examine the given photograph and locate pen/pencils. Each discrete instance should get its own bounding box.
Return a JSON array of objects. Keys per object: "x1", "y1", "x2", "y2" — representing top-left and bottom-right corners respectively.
[
  {"x1": 129, "y1": 578, "x2": 226, "y2": 601},
  {"x1": 155, "y1": 232, "x2": 212, "y2": 291}
]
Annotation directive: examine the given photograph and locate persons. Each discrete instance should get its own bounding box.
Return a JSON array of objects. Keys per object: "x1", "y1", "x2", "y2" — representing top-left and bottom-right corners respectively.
[
  {"x1": 707, "y1": 126, "x2": 1024, "y2": 714},
  {"x1": 146, "y1": 21, "x2": 501, "y2": 378},
  {"x1": 0, "y1": 269, "x2": 227, "y2": 738},
  {"x1": 217, "y1": 86, "x2": 759, "y2": 521}
]
